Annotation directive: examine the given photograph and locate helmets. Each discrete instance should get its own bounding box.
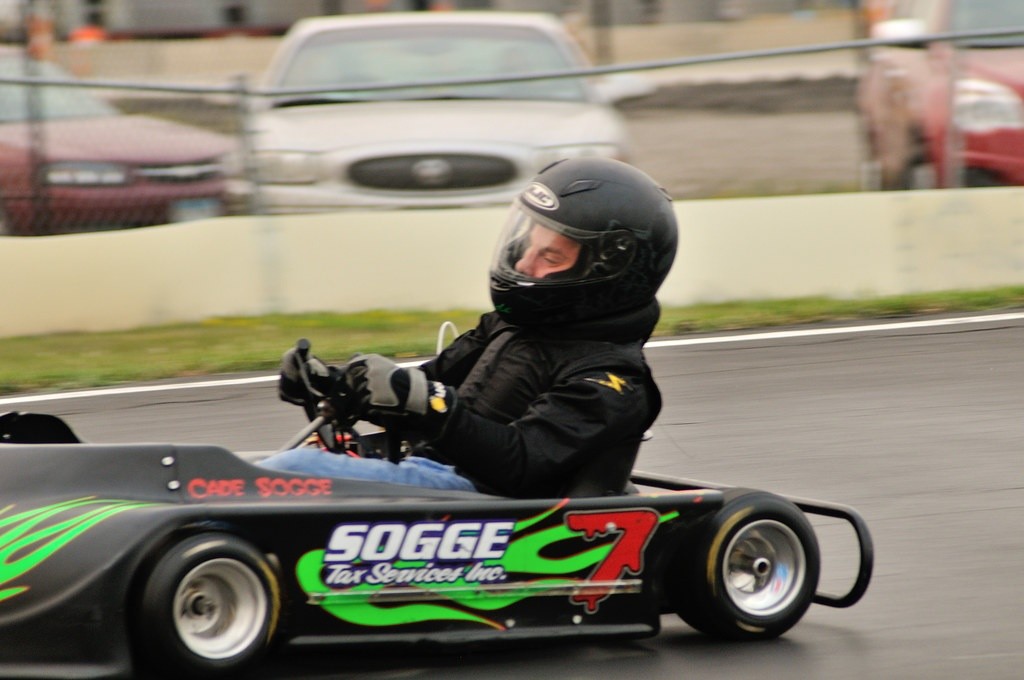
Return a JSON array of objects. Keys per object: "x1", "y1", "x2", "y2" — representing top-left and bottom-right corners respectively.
[{"x1": 488, "y1": 156, "x2": 679, "y2": 336}]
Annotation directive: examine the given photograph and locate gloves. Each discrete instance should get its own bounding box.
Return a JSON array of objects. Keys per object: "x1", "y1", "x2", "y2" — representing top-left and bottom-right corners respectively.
[
  {"x1": 277, "y1": 348, "x2": 340, "y2": 408},
  {"x1": 337, "y1": 354, "x2": 456, "y2": 424}
]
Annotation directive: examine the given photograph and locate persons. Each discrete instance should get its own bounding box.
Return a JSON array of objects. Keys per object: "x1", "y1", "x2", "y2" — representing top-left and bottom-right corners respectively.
[{"x1": 251, "y1": 154, "x2": 679, "y2": 497}]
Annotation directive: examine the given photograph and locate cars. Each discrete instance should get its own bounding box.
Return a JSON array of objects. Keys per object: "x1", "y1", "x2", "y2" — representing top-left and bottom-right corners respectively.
[
  {"x1": 1, "y1": 46, "x2": 242, "y2": 236},
  {"x1": 245, "y1": 10, "x2": 633, "y2": 214},
  {"x1": 849, "y1": 0, "x2": 1024, "y2": 190}
]
